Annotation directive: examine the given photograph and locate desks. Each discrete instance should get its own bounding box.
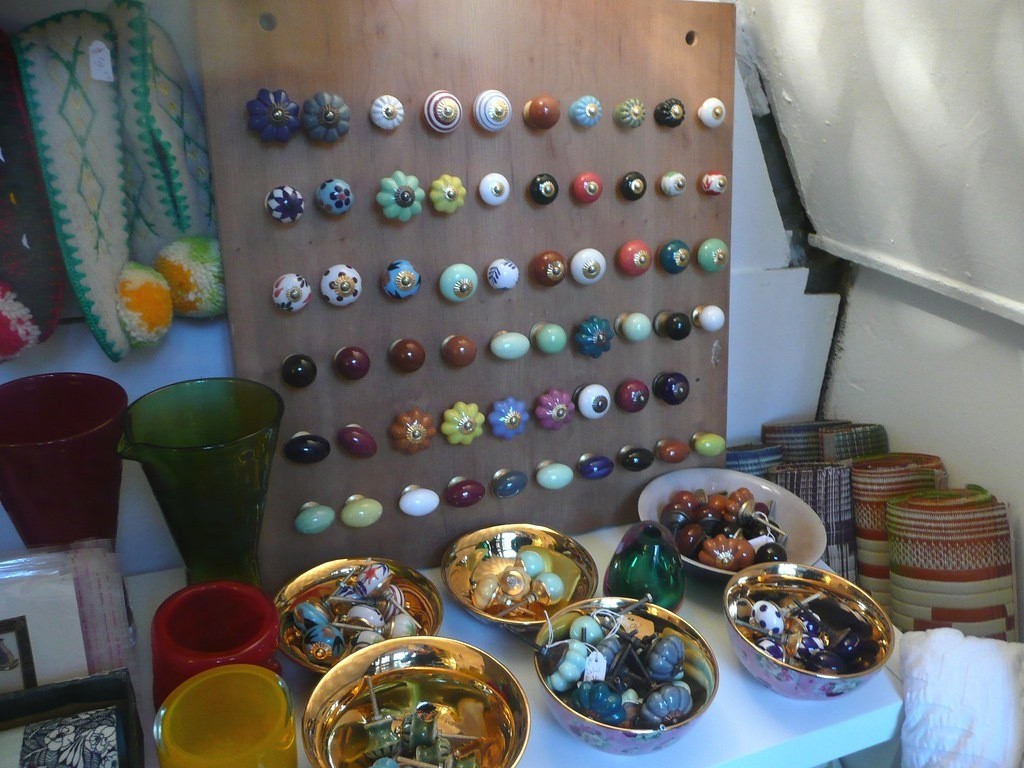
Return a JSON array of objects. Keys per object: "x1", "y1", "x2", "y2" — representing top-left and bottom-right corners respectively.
[{"x1": 276, "y1": 521, "x2": 906, "y2": 768}]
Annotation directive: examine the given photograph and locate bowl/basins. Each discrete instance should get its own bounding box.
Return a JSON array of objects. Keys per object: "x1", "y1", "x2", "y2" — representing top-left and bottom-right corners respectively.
[
  {"x1": 273, "y1": 554, "x2": 444, "y2": 676},
  {"x1": 536, "y1": 597, "x2": 721, "y2": 756},
  {"x1": 441, "y1": 523, "x2": 599, "y2": 634},
  {"x1": 722, "y1": 562, "x2": 896, "y2": 701},
  {"x1": 639, "y1": 468, "x2": 828, "y2": 587},
  {"x1": 152, "y1": 663, "x2": 297, "y2": 767},
  {"x1": 302, "y1": 635, "x2": 531, "y2": 768},
  {"x1": 146, "y1": 580, "x2": 284, "y2": 712}
]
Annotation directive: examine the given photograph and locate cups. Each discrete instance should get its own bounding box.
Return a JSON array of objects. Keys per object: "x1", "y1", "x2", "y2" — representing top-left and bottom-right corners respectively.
[
  {"x1": 117, "y1": 376, "x2": 285, "y2": 600},
  {"x1": 0, "y1": 372, "x2": 139, "y2": 650}
]
